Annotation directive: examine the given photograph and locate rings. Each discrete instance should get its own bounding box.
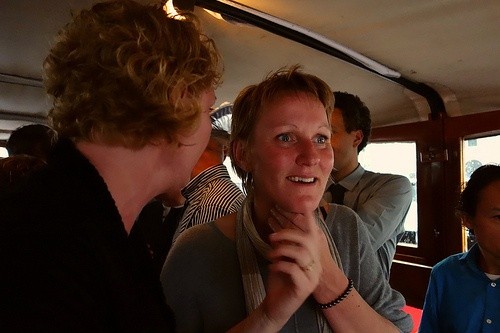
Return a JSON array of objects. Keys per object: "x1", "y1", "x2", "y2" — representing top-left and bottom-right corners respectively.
[{"x1": 300, "y1": 255, "x2": 317, "y2": 271}]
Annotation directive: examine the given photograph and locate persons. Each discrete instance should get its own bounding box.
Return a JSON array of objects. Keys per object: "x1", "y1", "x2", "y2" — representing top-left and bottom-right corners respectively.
[
  {"x1": 158, "y1": 62, "x2": 414, "y2": 333},
  {"x1": 0, "y1": 1, "x2": 226, "y2": 333},
  {"x1": 129, "y1": 187, "x2": 188, "y2": 279},
  {"x1": 5, "y1": 121, "x2": 60, "y2": 166},
  {"x1": 330, "y1": 91, "x2": 412, "y2": 284},
  {"x1": 168, "y1": 127, "x2": 247, "y2": 246},
  {"x1": 416, "y1": 164, "x2": 500, "y2": 333}
]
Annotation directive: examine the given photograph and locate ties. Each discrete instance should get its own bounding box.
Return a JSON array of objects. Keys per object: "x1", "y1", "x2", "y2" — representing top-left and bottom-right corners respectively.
[{"x1": 327, "y1": 183, "x2": 349, "y2": 205}]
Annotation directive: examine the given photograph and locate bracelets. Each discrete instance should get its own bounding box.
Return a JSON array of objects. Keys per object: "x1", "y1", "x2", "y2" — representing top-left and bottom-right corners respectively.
[{"x1": 315, "y1": 277, "x2": 354, "y2": 310}]
